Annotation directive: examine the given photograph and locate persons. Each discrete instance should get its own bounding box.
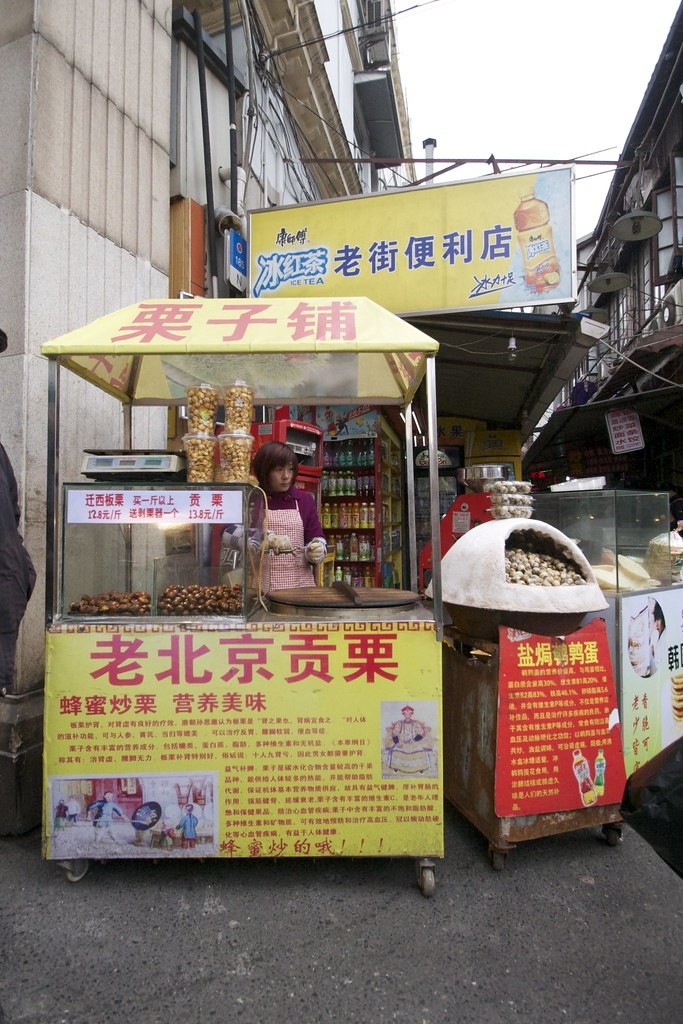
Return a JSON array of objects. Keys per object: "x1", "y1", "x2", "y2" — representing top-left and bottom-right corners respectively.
[
  {"x1": 1, "y1": 329, "x2": 38, "y2": 687},
  {"x1": 223, "y1": 440, "x2": 327, "y2": 595},
  {"x1": 649, "y1": 602, "x2": 666, "y2": 675}
]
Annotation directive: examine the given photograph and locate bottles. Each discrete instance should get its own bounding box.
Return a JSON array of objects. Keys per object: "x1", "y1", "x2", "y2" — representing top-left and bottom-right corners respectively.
[
  {"x1": 513, "y1": 186, "x2": 561, "y2": 295},
  {"x1": 322, "y1": 439, "x2": 400, "y2": 590},
  {"x1": 593, "y1": 749, "x2": 606, "y2": 797},
  {"x1": 572, "y1": 749, "x2": 597, "y2": 806},
  {"x1": 365, "y1": 421, "x2": 370, "y2": 436},
  {"x1": 324, "y1": 405, "x2": 337, "y2": 436},
  {"x1": 371, "y1": 422, "x2": 377, "y2": 436}
]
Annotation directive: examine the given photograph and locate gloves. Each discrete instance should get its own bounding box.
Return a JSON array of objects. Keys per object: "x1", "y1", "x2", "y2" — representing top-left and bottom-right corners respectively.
[
  {"x1": 306, "y1": 539, "x2": 328, "y2": 562},
  {"x1": 267, "y1": 534, "x2": 294, "y2": 555}
]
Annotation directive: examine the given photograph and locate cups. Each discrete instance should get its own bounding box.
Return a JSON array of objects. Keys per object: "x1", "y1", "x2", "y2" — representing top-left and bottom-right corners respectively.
[{"x1": 181, "y1": 379, "x2": 255, "y2": 483}]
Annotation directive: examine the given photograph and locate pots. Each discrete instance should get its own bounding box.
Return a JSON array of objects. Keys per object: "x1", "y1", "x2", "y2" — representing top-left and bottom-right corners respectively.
[{"x1": 424, "y1": 589, "x2": 610, "y2": 643}]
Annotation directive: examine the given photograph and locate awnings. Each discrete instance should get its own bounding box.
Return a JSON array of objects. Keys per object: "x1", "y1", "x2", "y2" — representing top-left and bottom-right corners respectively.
[{"x1": 400, "y1": 310, "x2": 683, "y2": 472}]
